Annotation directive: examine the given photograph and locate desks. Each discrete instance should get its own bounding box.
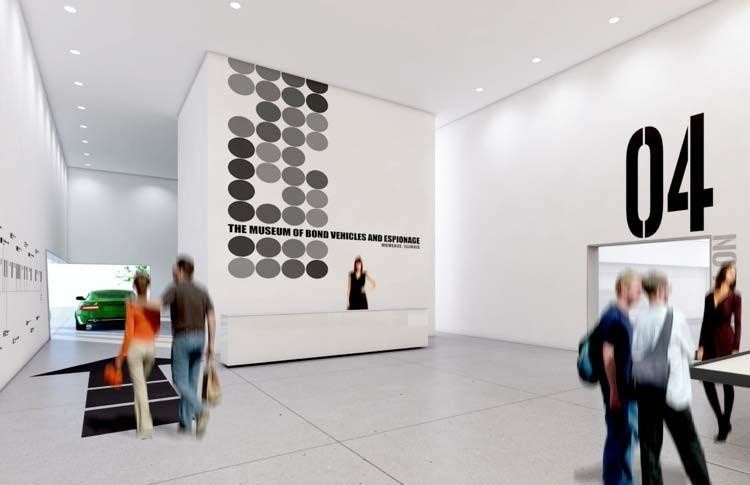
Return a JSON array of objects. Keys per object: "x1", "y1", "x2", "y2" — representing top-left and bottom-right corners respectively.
[{"x1": 690, "y1": 351, "x2": 750, "y2": 391}]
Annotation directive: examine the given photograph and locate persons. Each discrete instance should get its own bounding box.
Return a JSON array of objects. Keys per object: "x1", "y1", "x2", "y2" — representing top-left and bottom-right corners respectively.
[
  {"x1": 588, "y1": 267, "x2": 650, "y2": 484},
  {"x1": 693, "y1": 264, "x2": 744, "y2": 445},
  {"x1": 635, "y1": 271, "x2": 714, "y2": 485},
  {"x1": 110, "y1": 274, "x2": 162, "y2": 442},
  {"x1": 153, "y1": 257, "x2": 218, "y2": 439},
  {"x1": 346, "y1": 257, "x2": 377, "y2": 310}
]
